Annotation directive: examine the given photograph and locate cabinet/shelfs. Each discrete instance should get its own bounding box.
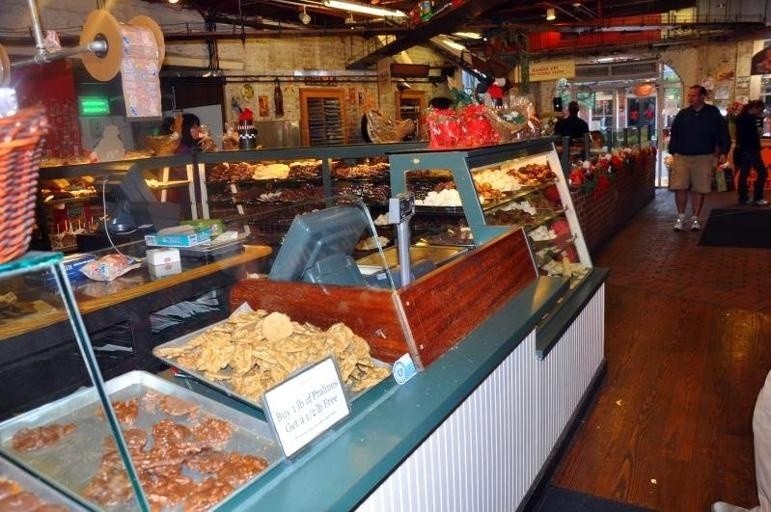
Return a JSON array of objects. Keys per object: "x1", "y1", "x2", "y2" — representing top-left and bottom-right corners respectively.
[{"x1": 1, "y1": 131, "x2": 611, "y2": 512}]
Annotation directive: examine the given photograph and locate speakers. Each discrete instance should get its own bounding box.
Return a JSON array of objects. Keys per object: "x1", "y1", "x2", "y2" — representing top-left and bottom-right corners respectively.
[{"x1": 553, "y1": 97, "x2": 561, "y2": 112}]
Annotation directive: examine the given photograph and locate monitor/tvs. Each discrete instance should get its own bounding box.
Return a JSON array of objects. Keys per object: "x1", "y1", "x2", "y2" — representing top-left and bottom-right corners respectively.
[
  {"x1": 267, "y1": 205, "x2": 368, "y2": 288},
  {"x1": 98, "y1": 163, "x2": 158, "y2": 231}
]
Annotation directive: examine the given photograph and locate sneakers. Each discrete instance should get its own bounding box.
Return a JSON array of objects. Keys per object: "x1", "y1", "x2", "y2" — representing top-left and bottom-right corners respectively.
[
  {"x1": 711, "y1": 500, "x2": 750, "y2": 512},
  {"x1": 755, "y1": 199, "x2": 769, "y2": 206},
  {"x1": 690, "y1": 218, "x2": 702, "y2": 231},
  {"x1": 740, "y1": 197, "x2": 754, "y2": 205},
  {"x1": 675, "y1": 216, "x2": 686, "y2": 231}
]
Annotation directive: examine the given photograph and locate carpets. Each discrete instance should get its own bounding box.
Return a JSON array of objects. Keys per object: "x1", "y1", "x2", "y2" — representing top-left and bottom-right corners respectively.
[{"x1": 697, "y1": 208, "x2": 771, "y2": 249}]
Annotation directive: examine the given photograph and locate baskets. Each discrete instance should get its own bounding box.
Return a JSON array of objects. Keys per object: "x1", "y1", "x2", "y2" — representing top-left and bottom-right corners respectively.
[{"x1": 0, "y1": 105, "x2": 52, "y2": 267}]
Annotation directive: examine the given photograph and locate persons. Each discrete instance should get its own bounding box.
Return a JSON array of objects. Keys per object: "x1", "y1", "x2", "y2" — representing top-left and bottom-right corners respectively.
[
  {"x1": 668, "y1": 85, "x2": 732, "y2": 232},
  {"x1": 731, "y1": 100, "x2": 771, "y2": 206},
  {"x1": 554, "y1": 101, "x2": 590, "y2": 146},
  {"x1": 710, "y1": 370, "x2": 771, "y2": 512},
  {"x1": 159, "y1": 116, "x2": 176, "y2": 134},
  {"x1": 176, "y1": 113, "x2": 203, "y2": 155}
]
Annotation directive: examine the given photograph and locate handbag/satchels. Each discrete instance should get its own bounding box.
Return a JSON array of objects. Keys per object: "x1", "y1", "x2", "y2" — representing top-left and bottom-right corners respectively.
[{"x1": 715, "y1": 162, "x2": 734, "y2": 193}]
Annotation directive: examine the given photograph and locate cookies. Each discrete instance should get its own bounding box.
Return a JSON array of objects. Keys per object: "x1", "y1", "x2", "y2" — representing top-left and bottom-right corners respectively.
[{"x1": 0, "y1": 309, "x2": 389, "y2": 512}]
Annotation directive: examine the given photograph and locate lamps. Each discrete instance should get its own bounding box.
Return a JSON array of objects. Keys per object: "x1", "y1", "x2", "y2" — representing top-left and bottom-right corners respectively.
[
  {"x1": 324, "y1": 0, "x2": 397, "y2": 18},
  {"x1": 547, "y1": 6, "x2": 556, "y2": 21}
]
